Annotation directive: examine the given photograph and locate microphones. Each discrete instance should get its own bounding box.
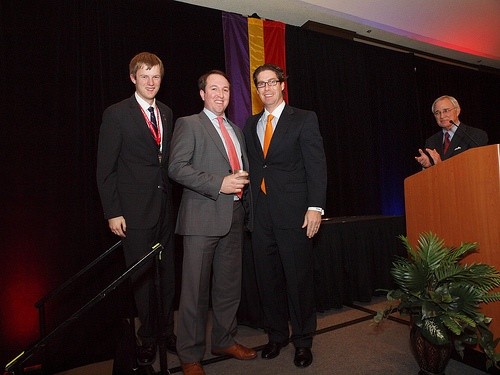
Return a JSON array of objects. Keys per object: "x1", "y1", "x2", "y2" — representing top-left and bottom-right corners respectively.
[{"x1": 449, "y1": 119, "x2": 479, "y2": 146}]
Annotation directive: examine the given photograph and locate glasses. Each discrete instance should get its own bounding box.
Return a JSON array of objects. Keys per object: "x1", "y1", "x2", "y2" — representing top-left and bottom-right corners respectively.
[
  {"x1": 433, "y1": 107, "x2": 456, "y2": 115},
  {"x1": 256, "y1": 78, "x2": 281, "y2": 88}
]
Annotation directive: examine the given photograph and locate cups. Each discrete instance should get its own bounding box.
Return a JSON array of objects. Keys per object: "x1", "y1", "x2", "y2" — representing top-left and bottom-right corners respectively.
[{"x1": 234, "y1": 170, "x2": 247, "y2": 180}]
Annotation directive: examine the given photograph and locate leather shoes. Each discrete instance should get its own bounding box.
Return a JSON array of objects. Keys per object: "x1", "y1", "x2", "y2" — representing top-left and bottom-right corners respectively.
[
  {"x1": 261, "y1": 339, "x2": 290, "y2": 360},
  {"x1": 182, "y1": 362, "x2": 207, "y2": 375},
  {"x1": 211, "y1": 342, "x2": 257, "y2": 360},
  {"x1": 293, "y1": 346, "x2": 313, "y2": 368}
]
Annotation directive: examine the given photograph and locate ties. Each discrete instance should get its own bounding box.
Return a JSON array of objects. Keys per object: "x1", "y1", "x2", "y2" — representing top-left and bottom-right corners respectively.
[
  {"x1": 442, "y1": 132, "x2": 451, "y2": 156},
  {"x1": 148, "y1": 106, "x2": 161, "y2": 150},
  {"x1": 261, "y1": 114, "x2": 274, "y2": 194},
  {"x1": 215, "y1": 116, "x2": 243, "y2": 200}
]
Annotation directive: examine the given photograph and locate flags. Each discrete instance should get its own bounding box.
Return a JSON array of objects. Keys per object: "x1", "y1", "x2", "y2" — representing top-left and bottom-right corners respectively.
[{"x1": 222, "y1": 11, "x2": 289, "y2": 134}]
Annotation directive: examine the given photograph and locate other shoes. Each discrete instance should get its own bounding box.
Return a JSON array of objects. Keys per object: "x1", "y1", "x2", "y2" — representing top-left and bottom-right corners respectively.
[
  {"x1": 162, "y1": 329, "x2": 178, "y2": 354},
  {"x1": 136, "y1": 340, "x2": 158, "y2": 365}
]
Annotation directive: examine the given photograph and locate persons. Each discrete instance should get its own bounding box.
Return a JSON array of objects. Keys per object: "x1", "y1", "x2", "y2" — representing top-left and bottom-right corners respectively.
[
  {"x1": 242, "y1": 63, "x2": 328, "y2": 367},
  {"x1": 95, "y1": 52, "x2": 178, "y2": 365},
  {"x1": 414, "y1": 96, "x2": 488, "y2": 170},
  {"x1": 169, "y1": 70, "x2": 258, "y2": 375}
]
